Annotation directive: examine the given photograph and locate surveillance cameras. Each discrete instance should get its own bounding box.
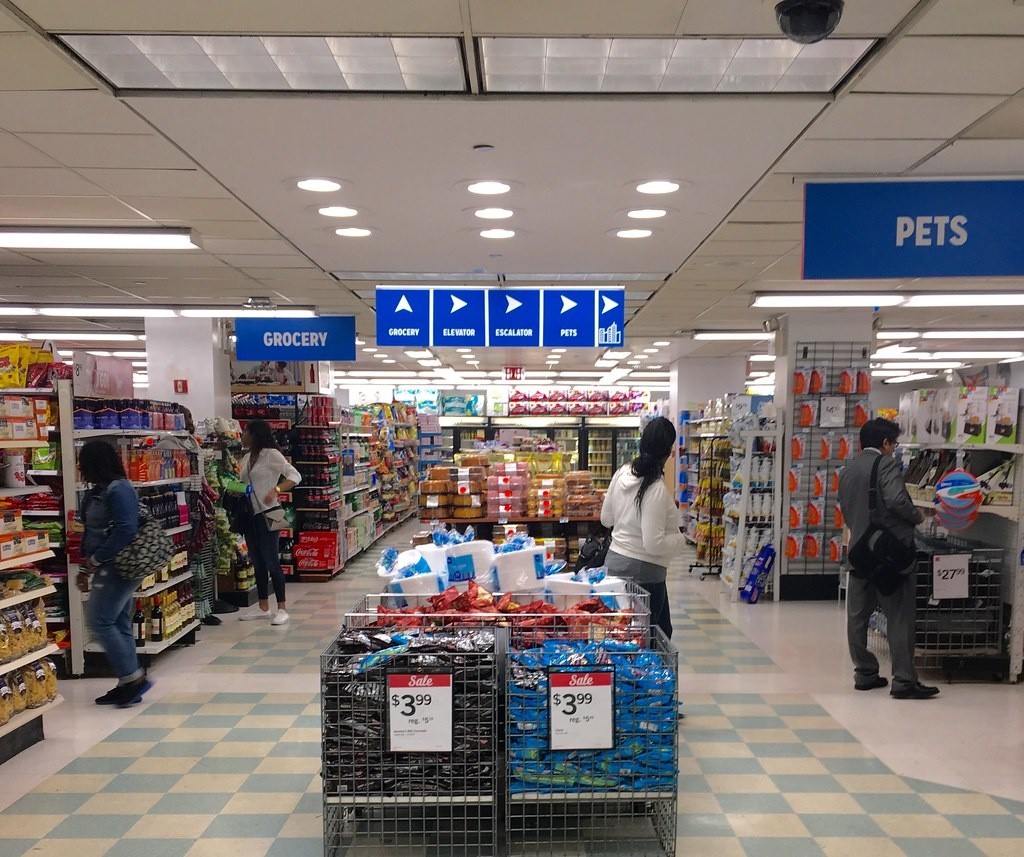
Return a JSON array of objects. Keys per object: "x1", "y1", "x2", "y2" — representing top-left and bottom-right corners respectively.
[{"x1": 774, "y1": 0, "x2": 844, "y2": 45}]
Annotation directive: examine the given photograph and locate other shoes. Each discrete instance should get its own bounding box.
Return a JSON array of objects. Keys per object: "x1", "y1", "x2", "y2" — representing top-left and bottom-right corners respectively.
[
  {"x1": 95, "y1": 685, "x2": 143, "y2": 705},
  {"x1": 116, "y1": 680, "x2": 151, "y2": 707},
  {"x1": 200, "y1": 615, "x2": 222, "y2": 625}
]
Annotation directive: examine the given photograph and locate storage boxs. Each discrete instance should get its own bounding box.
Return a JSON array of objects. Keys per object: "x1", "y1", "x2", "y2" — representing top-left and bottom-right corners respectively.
[{"x1": 896, "y1": 386, "x2": 1021, "y2": 444}]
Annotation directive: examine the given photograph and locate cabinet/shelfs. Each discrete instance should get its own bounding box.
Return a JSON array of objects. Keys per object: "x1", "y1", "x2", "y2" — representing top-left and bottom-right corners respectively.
[
  {"x1": 0, "y1": 379, "x2": 421, "y2": 766},
  {"x1": 682, "y1": 413, "x2": 767, "y2": 547},
  {"x1": 889, "y1": 442, "x2": 1022, "y2": 684}
]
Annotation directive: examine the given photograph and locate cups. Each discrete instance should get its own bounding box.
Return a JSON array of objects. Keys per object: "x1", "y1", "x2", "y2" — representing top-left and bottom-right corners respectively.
[{"x1": 2, "y1": 455, "x2": 26, "y2": 487}]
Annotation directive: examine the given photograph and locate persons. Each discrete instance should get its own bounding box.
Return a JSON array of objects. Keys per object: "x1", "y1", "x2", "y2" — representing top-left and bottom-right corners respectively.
[
  {"x1": 76, "y1": 440, "x2": 152, "y2": 710},
  {"x1": 837, "y1": 417, "x2": 940, "y2": 700},
  {"x1": 601, "y1": 416, "x2": 685, "y2": 719},
  {"x1": 238, "y1": 420, "x2": 302, "y2": 625}
]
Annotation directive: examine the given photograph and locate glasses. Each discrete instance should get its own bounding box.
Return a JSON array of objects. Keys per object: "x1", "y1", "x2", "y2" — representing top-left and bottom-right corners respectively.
[
  {"x1": 894, "y1": 440, "x2": 899, "y2": 447},
  {"x1": 76, "y1": 465, "x2": 81, "y2": 471}
]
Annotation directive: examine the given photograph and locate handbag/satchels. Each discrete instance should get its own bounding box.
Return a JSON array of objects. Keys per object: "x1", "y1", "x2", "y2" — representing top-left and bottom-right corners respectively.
[
  {"x1": 574, "y1": 524, "x2": 614, "y2": 574},
  {"x1": 103, "y1": 478, "x2": 176, "y2": 580},
  {"x1": 255, "y1": 505, "x2": 290, "y2": 534},
  {"x1": 848, "y1": 522, "x2": 918, "y2": 597}
]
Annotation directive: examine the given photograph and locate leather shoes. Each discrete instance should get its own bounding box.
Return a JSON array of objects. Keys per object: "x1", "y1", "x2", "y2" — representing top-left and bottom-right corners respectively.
[
  {"x1": 894, "y1": 682, "x2": 940, "y2": 698},
  {"x1": 855, "y1": 677, "x2": 888, "y2": 690}
]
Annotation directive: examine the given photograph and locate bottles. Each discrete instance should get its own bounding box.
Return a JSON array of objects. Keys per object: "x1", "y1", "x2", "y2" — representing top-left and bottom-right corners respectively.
[
  {"x1": 461, "y1": 431, "x2": 476, "y2": 440},
  {"x1": 588, "y1": 430, "x2": 642, "y2": 489},
  {"x1": 295, "y1": 430, "x2": 340, "y2": 508}
]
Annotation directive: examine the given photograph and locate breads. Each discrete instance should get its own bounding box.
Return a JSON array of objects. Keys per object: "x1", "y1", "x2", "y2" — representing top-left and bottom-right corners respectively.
[{"x1": 416, "y1": 456, "x2": 609, "y2": 518}]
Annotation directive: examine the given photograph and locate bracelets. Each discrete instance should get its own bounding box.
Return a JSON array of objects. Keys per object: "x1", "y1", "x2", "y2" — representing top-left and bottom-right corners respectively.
[{"x1": 275, "y1": 486, "x2": 281, "y2": 494}]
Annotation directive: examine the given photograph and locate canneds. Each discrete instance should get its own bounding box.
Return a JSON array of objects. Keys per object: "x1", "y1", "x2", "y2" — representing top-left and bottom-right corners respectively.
[
  {"x1": 307, "y1": 396, "x2": 333, "y2": 426},
  {"x1": 616, "y1": 429, "x2": 639, "y2": 465}
]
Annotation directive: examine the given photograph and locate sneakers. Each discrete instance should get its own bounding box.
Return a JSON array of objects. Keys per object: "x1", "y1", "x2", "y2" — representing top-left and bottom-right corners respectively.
[
  {"x1": 271, "y1": 609, "x2": 289, "y2": 625},
  {"x1": 239, "y1": 606, "x2": 271, "y2": 620}
]
